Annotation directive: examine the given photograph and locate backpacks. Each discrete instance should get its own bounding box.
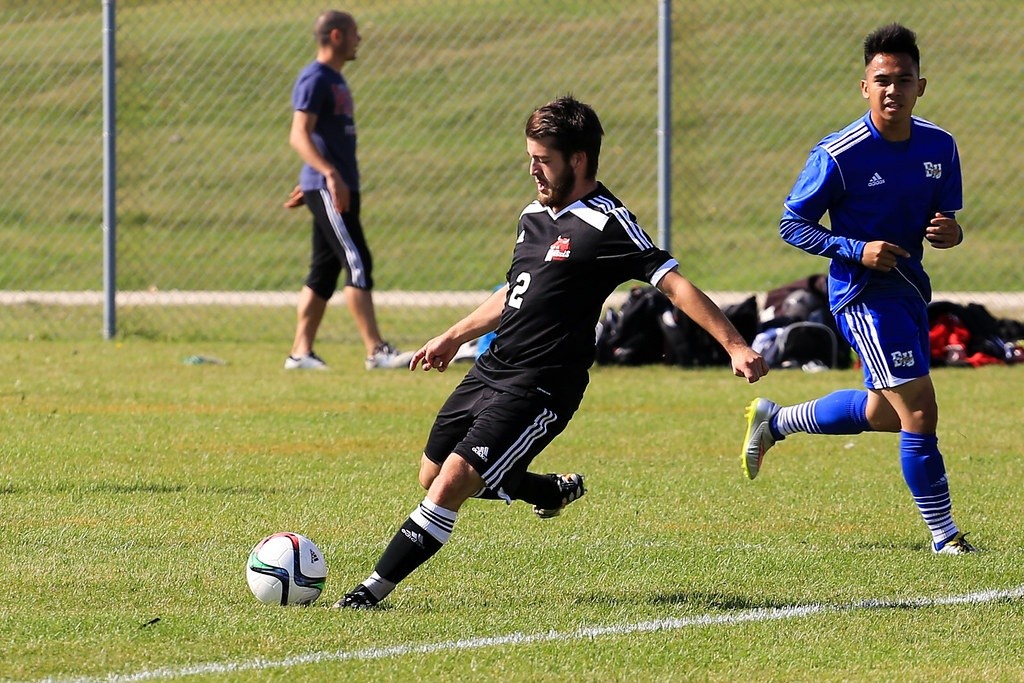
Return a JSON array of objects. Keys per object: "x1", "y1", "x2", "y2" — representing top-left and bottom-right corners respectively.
[{"x1": 595, "y1": 273, "x2": 853, "y2": 369}]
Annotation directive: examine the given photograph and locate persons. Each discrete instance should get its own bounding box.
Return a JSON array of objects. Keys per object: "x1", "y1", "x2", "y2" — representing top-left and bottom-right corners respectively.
[
  {"x1": 333, "y1": 94, "x2": 768, "y2": 607},
  {"x1": 742, "y1": 23, "x2": 976, "y2": 554},
  {"x1": 284, "y1": 10, "x2": 416, "y2": 370}
]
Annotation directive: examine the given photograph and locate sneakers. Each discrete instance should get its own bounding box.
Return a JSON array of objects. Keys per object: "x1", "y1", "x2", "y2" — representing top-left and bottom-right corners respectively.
[
  {"x1": 330, "y1": 590, "x2": 377, "y2": 611},
  {"x1": 365, "y1": 342, "x2": 418, "y2": 371},
  {"x1": 533, "y1": 472, "x2": 587, "y2": 518},
  {"x1": 929, "y1": 532, "x2": 976, "y2": 557},
  {"x1": 284, "y1": 351, "x2": 329, "y2": 370},
  {"x1": 741, "y1": 395, "x2": 784, "y2": 480}
]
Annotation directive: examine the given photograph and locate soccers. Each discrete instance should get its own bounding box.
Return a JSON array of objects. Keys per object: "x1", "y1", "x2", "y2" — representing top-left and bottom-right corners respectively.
[{"x1": 244, "y1": 530, "x2": 331, "y2": 607}]
